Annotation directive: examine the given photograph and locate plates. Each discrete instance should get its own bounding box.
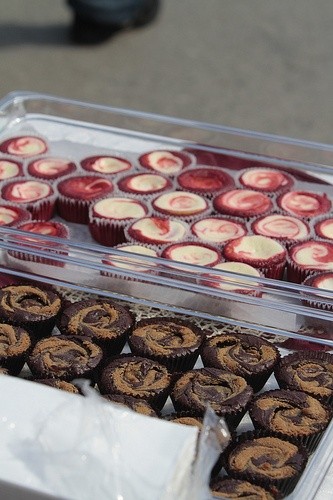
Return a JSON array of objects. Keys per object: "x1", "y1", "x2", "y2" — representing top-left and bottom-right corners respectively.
[
  {"x1": 1, "y1": 90, "x2": 333, "y2": 309},
  {"x1": 0, "y1": 241, "x2": 333, "y2": 500}
]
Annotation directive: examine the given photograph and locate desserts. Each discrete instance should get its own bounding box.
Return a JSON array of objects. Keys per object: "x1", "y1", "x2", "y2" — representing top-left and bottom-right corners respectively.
[
  {"x1": 0, "y1": 285, "x2": 333, "y2": 500},
  {"x1": 0, "y1": 133, "x2": 333, "y2": 332}
]
